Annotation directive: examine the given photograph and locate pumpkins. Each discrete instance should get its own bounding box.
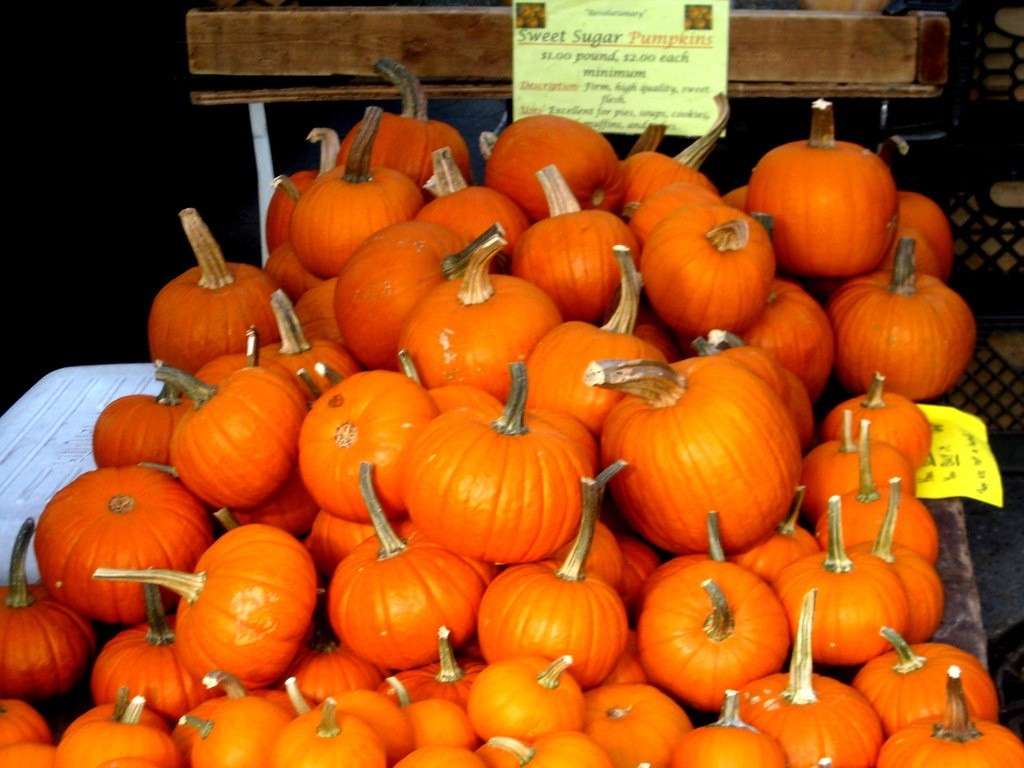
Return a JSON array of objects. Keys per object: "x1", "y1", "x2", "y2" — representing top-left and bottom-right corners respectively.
[{"x1": 0, "y1": 56, "x2": 1024, "y2": 768}]
